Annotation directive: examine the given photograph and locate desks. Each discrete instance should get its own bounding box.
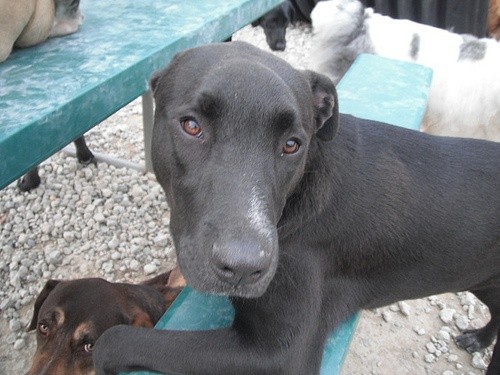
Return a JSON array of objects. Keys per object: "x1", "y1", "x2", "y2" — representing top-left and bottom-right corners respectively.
[{"x1": 1, "y1": 0, "x2": 287, "y2": 209}]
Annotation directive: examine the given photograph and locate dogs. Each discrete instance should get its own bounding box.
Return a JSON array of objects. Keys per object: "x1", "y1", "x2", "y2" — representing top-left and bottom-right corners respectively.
[
  {"x1": 251, "y1": 0, "x2": 317, "y2": 52},
  {"x1": 90, "y1": 41, "x2": 500, "y2": 375},
  {"x1": 24, "y1": 270, "x2": 184, "y2": 375},
  {"x1": 307, "y1": 0, "x2": 500, "y2": 144},
  {"x1": 0, "y1": 0, "x2": 85, "y2": 66}
]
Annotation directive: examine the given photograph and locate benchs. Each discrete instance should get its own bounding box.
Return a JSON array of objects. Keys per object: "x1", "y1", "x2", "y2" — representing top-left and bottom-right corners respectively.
[{"x1": 121, "y1": 55, "x2": 435, "y2": 375}]
